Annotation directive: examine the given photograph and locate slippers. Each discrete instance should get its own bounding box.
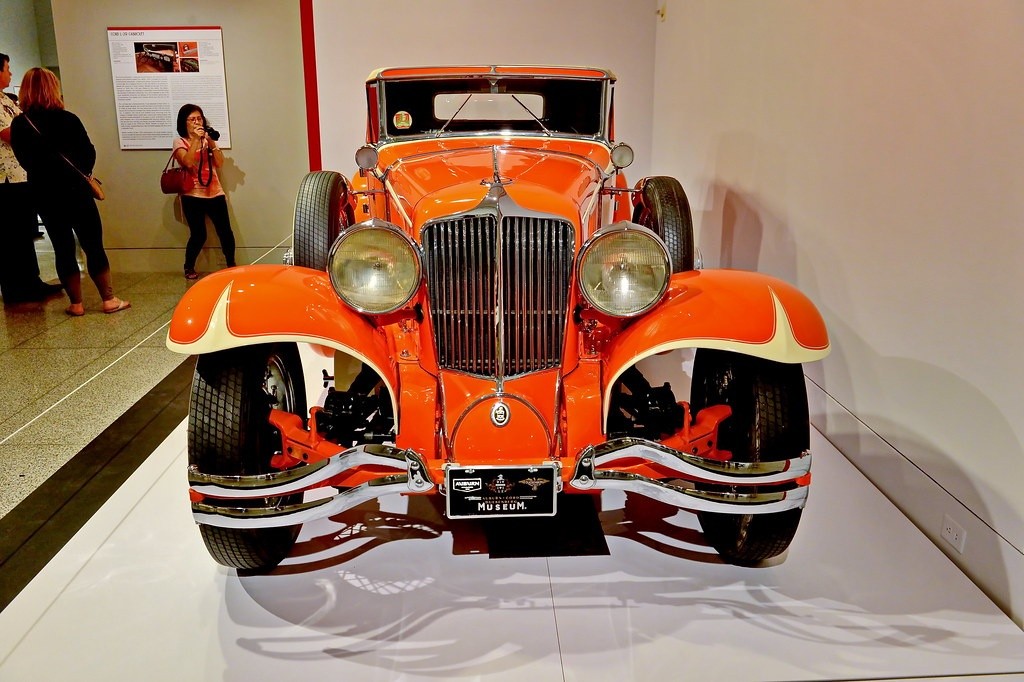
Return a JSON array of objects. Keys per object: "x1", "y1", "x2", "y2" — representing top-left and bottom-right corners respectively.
[
  {"x1": 104, "y1": 299, "x2": 131, "y2": 314},
  {"x1": 64, "y1": 306, "x2": 85, "y2": 316}
]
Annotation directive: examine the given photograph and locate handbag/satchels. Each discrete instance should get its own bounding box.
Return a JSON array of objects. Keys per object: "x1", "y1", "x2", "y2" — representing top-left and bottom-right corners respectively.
[
  {"x1": 87, "y1": 176, "x2": 105, "y2": 201},
  {"x1": 161, "y1": 148, "x2": 195, "y2": 194}
]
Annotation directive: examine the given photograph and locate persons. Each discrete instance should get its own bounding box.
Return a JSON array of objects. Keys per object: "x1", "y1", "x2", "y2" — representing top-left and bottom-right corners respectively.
[
  {"x1": 10, "y1": 67, "x2": 132, "y2": 316},
  {"x1": 172, "y1": 104, "x2": 236, "y2": 280},
  {"x1": 0, "y1": 53, "x2": 64, "y2": 305}
]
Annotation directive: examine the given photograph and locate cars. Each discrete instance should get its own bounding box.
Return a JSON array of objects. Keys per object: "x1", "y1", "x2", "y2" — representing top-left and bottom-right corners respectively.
[{"x1": 166, "y1": 66, "x2": 832, "y2": 570}]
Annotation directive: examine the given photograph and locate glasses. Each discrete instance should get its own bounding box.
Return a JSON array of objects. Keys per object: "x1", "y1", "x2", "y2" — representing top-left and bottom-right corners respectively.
[{"x1": 187, "y1": 117, "x2": 204, "y2": 124}]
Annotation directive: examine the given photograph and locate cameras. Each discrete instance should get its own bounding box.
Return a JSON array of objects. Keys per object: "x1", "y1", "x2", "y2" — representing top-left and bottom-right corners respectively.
[{"x1": 200, "y1": 126, "x2": 221, "y2": 141}]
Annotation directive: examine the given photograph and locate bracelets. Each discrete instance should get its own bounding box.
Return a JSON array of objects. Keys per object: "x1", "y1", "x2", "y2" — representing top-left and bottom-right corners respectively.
[{"x1": 212, "y1": 147, "x2": 220, "y2": 151}]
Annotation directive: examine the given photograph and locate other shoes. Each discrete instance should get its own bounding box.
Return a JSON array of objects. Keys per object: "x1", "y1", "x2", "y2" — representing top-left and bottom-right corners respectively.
[
  {"x1": 3, "y1": 277, "x2": 63, "y2": 304},
  {"x1": 185, "y1": 271, "x2": 199, "y2": 279},
  {"x1": 36, "y1": 231, "x2": 45, "y2": 237}
]
